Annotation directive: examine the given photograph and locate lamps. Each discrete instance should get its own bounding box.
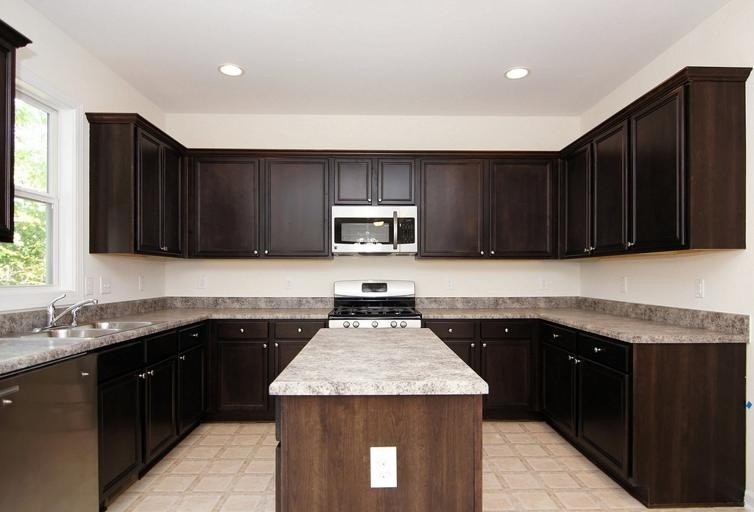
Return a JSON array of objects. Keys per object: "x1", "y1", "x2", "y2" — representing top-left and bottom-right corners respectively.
[
  {"x1": 504, "y1": 67, "x2": 528, "y2": 79},
  {"x1": 218, "y1": 65, "x2": 243, "y2": 77}
]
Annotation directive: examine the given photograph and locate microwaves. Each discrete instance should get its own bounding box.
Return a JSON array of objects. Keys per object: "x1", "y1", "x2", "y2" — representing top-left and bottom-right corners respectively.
[{"x1": 331, "y1": 205, "x2": 417, "y2": 256}]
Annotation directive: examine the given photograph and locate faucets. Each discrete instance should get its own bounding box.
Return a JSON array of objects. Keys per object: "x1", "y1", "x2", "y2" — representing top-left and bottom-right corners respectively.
[{"x1": 30, "y1": 293, "x2": 98, "y2": 334}]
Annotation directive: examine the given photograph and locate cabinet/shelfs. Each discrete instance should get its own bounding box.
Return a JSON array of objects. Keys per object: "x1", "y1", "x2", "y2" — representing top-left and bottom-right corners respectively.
[
  {"x1": 177, "y1": 321, "x2": 205, "y2": 437},
  {"x1": 186, "y1": 148, "x2": 327, "y2": 259},
  {"x1": 630, "y1": 83, "x2": 683, "y2": 253},
  {"x1": 205, "y1": 318, "x2": 327, "y2": 421},
  {"x1": 531, "y1": 319, "x2": 577, "y2": 439},
  {"x1": 557, "y1": 137, "x2": 589, "y2": 250},
  {"x1": 421, "y1": 155, "x2": 553, "y2": 259},
  {"x1": 576, "y1": 328, "x2": 632, "y2": 482},
  {"x1": 426, "y1": 318, "x2": 538, "y2": 422},
  {"x1": 134, "y1": 112, "x2": 184, "y2": 255},
  {"x1": 143, "y1": 330, "x2": 177, "y2": 473},
  {"x1": 95, "y1": 342, "x2": 142, "y2": 505},
  {"x1": 592, "y1": 119, "x2": 630, "y2": 256},
  {"x1": 330, "y1": 154, "x2": 416, "y2": 205}
]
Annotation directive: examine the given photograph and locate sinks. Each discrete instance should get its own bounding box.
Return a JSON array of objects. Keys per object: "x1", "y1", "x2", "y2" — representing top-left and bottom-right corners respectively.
[
  {"x1": 71, "y1": 320, "x2": 168, "y2": 331},
  {"x1": 21, "y1": 327, "x2": 120, "y2": 339}
]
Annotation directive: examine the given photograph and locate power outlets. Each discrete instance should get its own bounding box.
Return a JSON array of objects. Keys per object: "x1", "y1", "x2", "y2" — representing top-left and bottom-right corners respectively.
[{"x1": 369, "y1": 446, "x2": 398, "y2": 487}]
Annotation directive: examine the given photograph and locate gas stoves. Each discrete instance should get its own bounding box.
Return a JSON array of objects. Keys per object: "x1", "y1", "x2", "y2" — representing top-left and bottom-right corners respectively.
[{"x1": 327, "y1": 281, "x2": 422, "y2": 329}]
